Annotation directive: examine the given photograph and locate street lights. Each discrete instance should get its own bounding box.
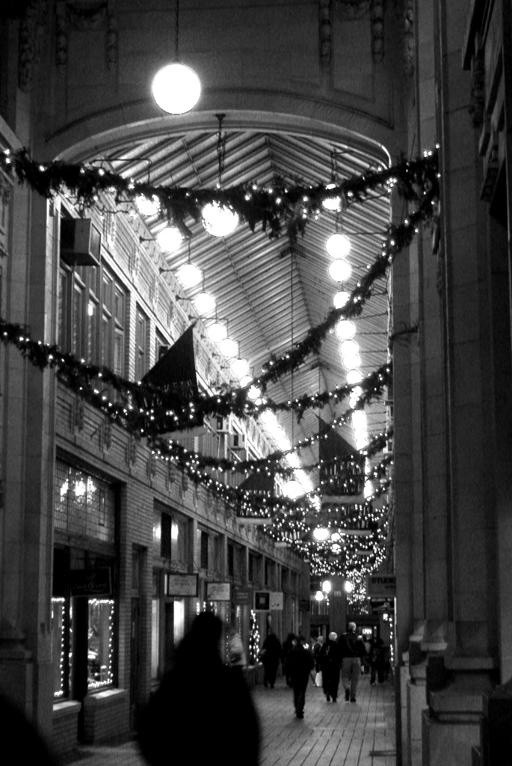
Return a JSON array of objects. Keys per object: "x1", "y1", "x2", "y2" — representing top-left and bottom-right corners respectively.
[{"x1": 314, "y1": 671, "x2": 323, "y2": 687}]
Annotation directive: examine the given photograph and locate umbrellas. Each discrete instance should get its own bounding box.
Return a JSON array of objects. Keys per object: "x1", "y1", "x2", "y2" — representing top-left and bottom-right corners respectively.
[{"x1": 327, "y1": 691, "x2": 337, "y2": 702}]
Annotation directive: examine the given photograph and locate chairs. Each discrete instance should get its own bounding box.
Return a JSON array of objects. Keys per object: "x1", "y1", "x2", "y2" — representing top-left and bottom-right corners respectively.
[{"x1": 345, "y1": 688, "x2": 356, "y2": 702}]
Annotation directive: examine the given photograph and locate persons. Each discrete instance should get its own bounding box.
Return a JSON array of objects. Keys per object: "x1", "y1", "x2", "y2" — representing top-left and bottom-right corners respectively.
[{"x1": 132, "y1": 610, "x2": 390, "y2": 764}]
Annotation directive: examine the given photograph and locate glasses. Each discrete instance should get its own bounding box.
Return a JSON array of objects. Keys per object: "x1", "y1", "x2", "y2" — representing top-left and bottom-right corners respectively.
[
  {"x1": 133, "y1": 156, "x2": 254, "y2": 388},
  {"x1": 200, "y1": 130, "x2": 241, "y2": 236},
  {"x1": 321, "y1": 148, "x2": 350, "y2": 311},
  {"x1": 149, "y1": 0, "x2": 203, "y2": 114}
]
